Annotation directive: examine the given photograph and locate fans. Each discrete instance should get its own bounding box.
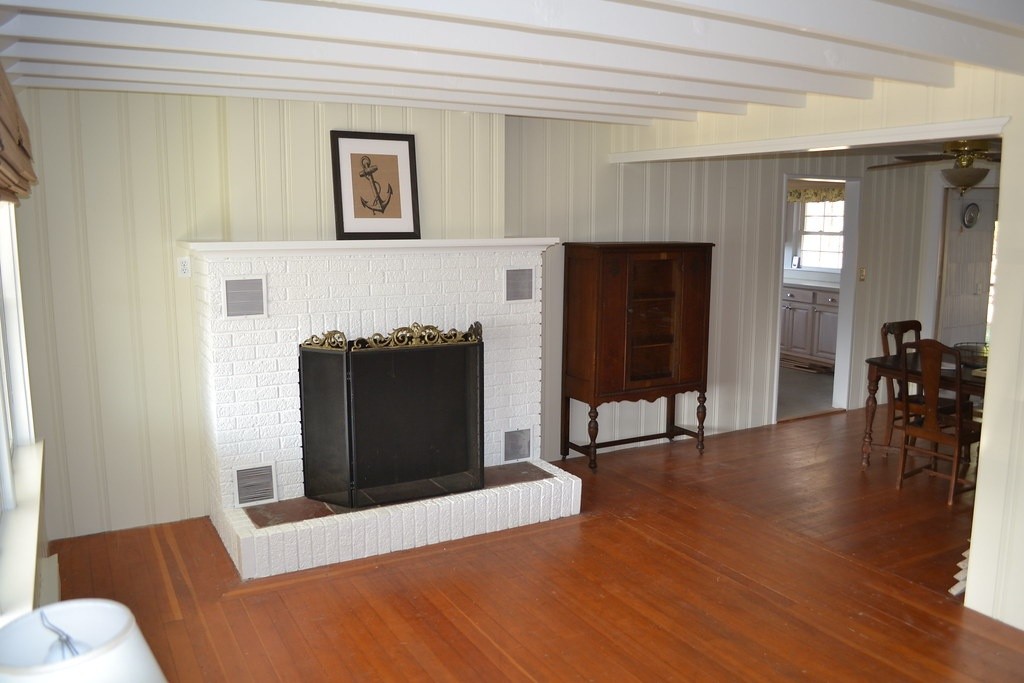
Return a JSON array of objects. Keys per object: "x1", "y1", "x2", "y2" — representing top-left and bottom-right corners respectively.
[{"x1": 866, "y1": 139, "x2": 1001, "y2": 170}]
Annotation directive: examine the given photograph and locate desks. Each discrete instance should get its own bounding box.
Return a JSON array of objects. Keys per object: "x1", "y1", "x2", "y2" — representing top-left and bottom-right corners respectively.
[{"x1": 860, "y1": 347, "x2": 988, "y2": 472}]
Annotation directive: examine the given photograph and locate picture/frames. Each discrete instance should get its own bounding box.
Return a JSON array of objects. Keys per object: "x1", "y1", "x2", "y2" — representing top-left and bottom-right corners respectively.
[{"x1": 330, "y1": 130, "x2": 421, "y2": 240}]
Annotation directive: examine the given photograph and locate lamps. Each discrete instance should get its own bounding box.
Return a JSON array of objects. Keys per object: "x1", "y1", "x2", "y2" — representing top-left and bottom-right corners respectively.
[
  {"x1": 940, "y1": 156, "x2": 989, "y2": 197},
  {"x1": 0, "y1": 598, "x2": 168, "y2": 683}
]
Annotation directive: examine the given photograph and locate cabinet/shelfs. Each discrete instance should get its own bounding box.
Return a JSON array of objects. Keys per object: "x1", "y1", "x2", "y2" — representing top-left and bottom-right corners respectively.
[
  {"x1": 562, "y1": 240, "x2": 717, "y2": 472},
  {"x1": 780, "y1": 287, "x2": 839, "y2": 373}
]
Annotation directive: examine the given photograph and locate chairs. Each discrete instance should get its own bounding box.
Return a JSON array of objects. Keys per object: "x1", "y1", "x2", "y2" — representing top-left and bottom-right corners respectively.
[
  {"x1": 882, "y1": 320, "x2": 973, "y2": 458},
  {"x1": 895, "y1": 339, "x2": 982, "y2": 506}
]
